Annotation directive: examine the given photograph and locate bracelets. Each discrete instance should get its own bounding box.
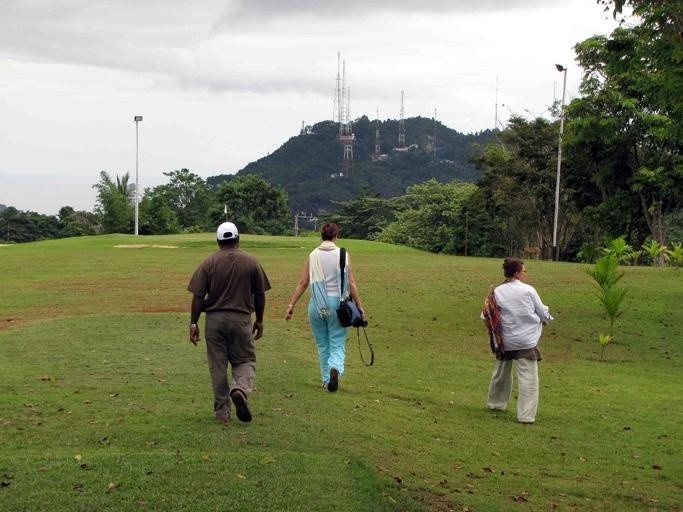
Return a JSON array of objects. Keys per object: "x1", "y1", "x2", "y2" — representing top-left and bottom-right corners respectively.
[
  {"x1": 287, "y1": 304, "x2": 296, "y2": 308},
  {"x1": 190, "y1": 323, "x2": 197, "y2": 330}
]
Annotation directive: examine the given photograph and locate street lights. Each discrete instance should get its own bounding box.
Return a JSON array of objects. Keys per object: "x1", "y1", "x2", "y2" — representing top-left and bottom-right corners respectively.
[
  {"x1": 552, "y1": 63, "x2": 567, "y2": 260},
  {"x1": 133, "y1": 116, "x2": 142, "y2": 234}
]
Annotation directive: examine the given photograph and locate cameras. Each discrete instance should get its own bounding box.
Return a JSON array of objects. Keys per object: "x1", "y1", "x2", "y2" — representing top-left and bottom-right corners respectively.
[{"x1": 355, "y1": 319, "x2": 368, "y2": 329}]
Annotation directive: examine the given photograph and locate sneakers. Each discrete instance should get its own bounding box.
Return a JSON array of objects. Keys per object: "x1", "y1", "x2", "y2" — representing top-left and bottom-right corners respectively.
[
  {"x1": 231, "y1": 389, "x2": 252, "y2": 423},
  {"x1": 328, "y1": 368, "x2": 340, "y2": 393}
]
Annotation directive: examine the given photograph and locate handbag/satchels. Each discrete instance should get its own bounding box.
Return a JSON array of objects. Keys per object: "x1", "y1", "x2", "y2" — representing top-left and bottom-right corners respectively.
[{"x1": 336, "y1": 300, "x2": 361, "y2": 327}]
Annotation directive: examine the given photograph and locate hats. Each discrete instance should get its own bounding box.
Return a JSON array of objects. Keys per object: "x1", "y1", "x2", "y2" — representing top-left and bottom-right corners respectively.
[{"x1": 217, "y1": 222, "x2": 239, "y2": 241}]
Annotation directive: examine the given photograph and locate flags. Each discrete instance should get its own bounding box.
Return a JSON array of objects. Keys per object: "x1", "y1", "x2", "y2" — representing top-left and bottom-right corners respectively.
[{"x1": 223, "y1": 205, "x2": 227, "y2": 214}]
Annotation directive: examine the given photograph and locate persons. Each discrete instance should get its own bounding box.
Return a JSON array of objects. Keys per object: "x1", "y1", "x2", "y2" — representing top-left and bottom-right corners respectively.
[
  {"x1": 480, "y1": 257, "x2": 555, "y2": 424},
  {"x1": 187, "y1": 222, "x2": 273, "y2": 426},
  {"x1": 285, "y1": 223, "x2": 366, "y2": 392}
]
